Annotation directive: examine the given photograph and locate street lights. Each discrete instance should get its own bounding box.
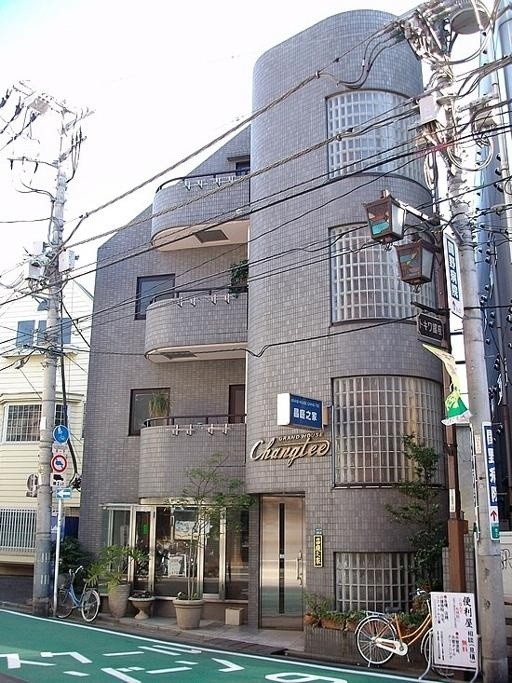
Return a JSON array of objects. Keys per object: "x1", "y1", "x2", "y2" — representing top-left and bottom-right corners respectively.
[{"x1": 362, "y1": 190, "x2": 468, "y2": 593}]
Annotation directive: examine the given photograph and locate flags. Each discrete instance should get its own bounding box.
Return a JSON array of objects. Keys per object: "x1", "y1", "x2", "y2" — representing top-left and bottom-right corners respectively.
[{"x1": 422, "y1": 343, "x2": 473, "y2": 426}]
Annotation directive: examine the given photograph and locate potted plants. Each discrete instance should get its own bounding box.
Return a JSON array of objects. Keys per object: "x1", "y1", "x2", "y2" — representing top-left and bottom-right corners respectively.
[
  {"x1": 83, "y1": 454, "x2": 250, "y2": 631},
  {"x1": 300, "y1": 587, "x2": 430, "y2": 641}
]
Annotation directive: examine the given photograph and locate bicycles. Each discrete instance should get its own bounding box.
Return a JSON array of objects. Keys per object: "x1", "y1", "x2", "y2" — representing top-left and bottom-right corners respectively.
[
  {"x1": 354, "y1": 587, "x2": 454, "y2": 678},
  {"x1": 50, "y1": 566, "x2": 101, "y2": 624}
]
